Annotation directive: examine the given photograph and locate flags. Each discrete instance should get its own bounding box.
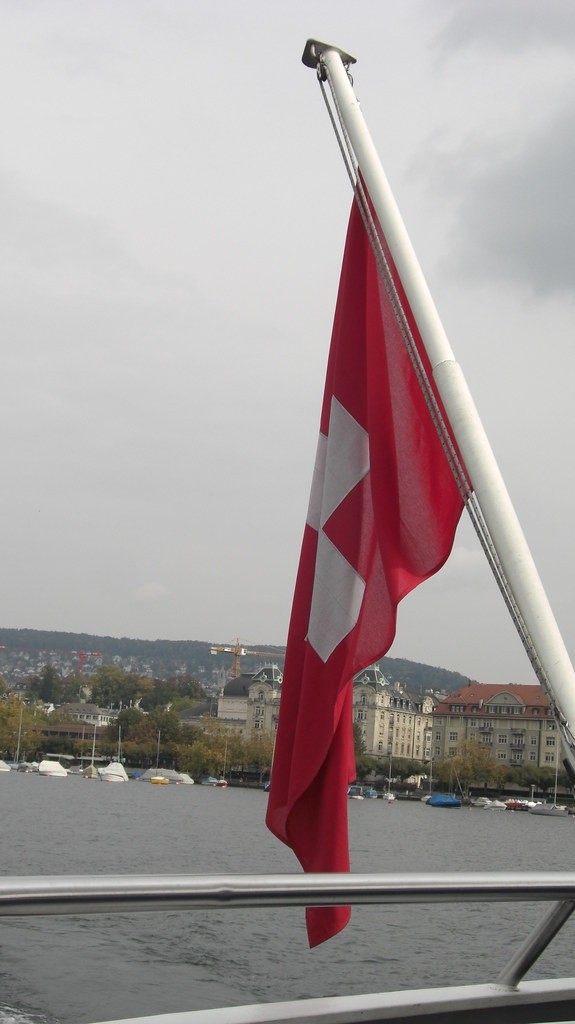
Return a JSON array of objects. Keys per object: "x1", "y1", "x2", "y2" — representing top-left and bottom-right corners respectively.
[{"x1": 266, "y1": 166, "x2": 474, "y2": 950}]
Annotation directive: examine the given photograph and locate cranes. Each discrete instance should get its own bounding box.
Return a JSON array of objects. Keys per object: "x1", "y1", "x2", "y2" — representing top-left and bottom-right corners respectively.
[
  {"x1": 209, "y1": 636, "x2": 286, "y2": 680},
  {"x1": 0, "y1": 643, "x2": 103, "y2": 680}
]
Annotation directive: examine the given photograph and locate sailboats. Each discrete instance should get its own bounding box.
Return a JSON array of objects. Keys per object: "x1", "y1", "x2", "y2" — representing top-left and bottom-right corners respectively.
[{"x1": 0, "y1": 704, "x2": 570, "y2": 818}]
